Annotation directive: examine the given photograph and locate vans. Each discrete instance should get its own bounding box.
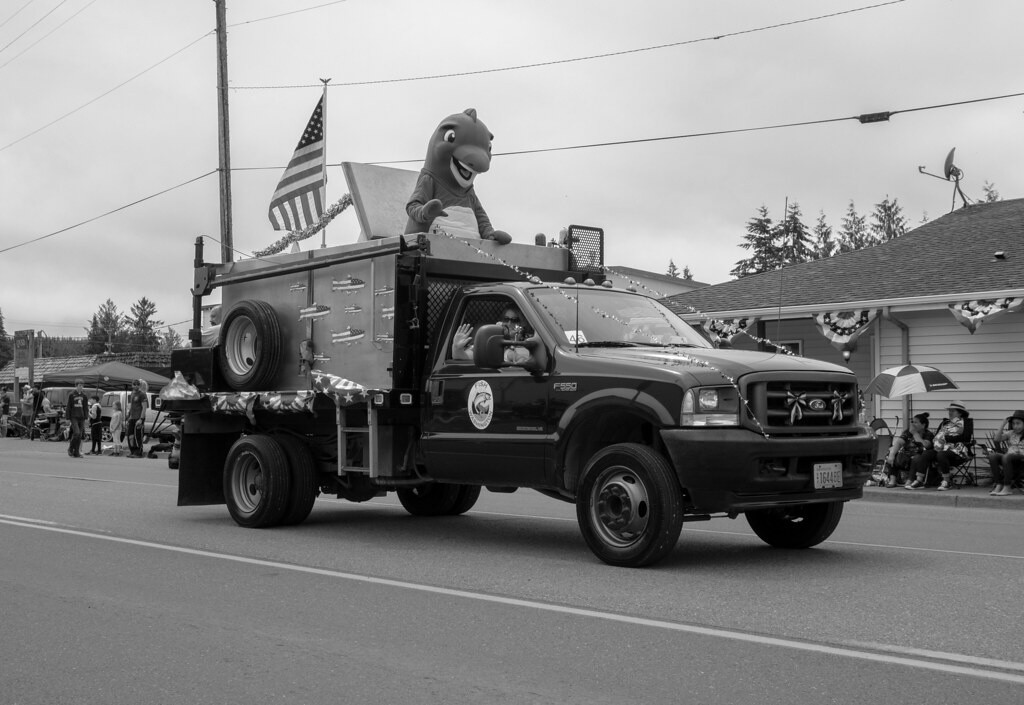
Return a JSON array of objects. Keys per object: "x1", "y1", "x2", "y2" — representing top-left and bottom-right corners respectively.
[{"x1": 44, "y1": 387, "x2": 106, "y2": 412}]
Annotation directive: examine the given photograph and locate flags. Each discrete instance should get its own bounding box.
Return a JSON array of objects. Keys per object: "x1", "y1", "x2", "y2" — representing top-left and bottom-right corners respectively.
[{"x1": 269, "y1": 92, "x2": 327, "y2": 232}]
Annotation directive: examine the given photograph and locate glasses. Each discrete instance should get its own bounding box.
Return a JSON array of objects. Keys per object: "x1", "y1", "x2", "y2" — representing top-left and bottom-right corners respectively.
[
  {"x1": 500, "y1": 316, "x2": 522, "y2": 324},
  {"x1": 132, "y1": 383, "x2": 140, "y2": 386}
]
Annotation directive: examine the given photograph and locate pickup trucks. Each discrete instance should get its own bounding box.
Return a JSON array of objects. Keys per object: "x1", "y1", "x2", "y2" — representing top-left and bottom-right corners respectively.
[
  {"x1": 98, "y1": 390, "x2": 179, "y2": 443},
  {"x1": 151, "y1": 225, "x2": 878, "y2": 569}
]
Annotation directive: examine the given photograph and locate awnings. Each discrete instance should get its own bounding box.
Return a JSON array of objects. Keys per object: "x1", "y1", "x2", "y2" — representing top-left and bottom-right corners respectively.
[{"x1": 42, "y1": 361, "x2": 173, "y2": 396}]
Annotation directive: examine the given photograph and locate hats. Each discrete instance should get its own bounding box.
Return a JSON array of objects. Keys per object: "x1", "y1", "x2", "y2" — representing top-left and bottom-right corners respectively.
[
  {"x1": 1006, "y1": 410, "x2": 1024, "y2": 422},
  {"x1": 944, "y1": 400, "x2": 969, "y2": 418},
  {"x1": 21, "y1": 385, "x2": 31, "y2": 390},
  {"x1": 31, "y1": 384, "x2": 41, "y2": 390}
]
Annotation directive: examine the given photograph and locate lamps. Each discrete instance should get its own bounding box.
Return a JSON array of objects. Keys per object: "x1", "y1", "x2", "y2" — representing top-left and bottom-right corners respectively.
[
  {"x1": 842, "y1": 341, "x2": 857, "y2": 364},
  {"x1": 994, "y1": 251, "x2": 1007, "y2": 259}
]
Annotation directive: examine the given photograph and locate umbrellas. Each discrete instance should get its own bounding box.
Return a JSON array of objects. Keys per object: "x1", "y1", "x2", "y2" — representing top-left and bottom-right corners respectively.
[{"x1": 863, "y1": 360, "x2": 960, "y2": 419}]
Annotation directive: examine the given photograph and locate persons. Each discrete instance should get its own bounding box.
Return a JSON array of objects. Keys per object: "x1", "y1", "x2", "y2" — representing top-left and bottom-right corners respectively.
[
  {"x1": 0, "y1": 385, "x2": 55, "y2": 440},
  {"x1": 108, "y1": 401, "x2": 122, "y2": 456},
  {"x1": 84, "y1": 395, "x2": 102, "y2": 455},
  {"x1": 125, "y1": 378, "x2": 149, "y2": 458},
  {"x1": 452, "y1": 303, "x2": 530, "y2": 362},
  {"x1": 904, "y1": 401, "x2": 974, "y2": 491},
  {"x1": 64, "y1": 379, "x2": 89, "y2": 458},
  {"x1": 989, "y1": 410, "x2": 1024, "y2": 496},
  {"x1": 886, "y1": 412, "x2": 935, "y2": 487}
]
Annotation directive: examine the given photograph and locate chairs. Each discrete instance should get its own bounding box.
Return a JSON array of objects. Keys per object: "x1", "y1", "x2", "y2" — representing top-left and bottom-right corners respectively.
[{"x1": 929, "y1": 417, "x2": 978, "y2": 489}]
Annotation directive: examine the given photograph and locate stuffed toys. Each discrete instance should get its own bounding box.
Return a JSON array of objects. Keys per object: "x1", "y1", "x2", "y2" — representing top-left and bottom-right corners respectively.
[{"x1": 404, "y1": 108, "x2": 511, "y2": 245}]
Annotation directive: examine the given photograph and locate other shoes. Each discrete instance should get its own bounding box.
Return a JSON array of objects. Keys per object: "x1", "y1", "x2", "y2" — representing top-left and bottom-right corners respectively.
[
  {"x1": 95, "y1": 451, "x2": 102, "y2": 455},
  {"x1": 127, "y1": 451, "x2": 134, "y2": 457},
  {"x1": 86, "y1": 451, "x2": 95, "y2": 455},
  {"x1": 886, "y1": 482, "x2": 898, "y2": 488},
  {"x1": 988, "y1": 491, "x2": 999, "y2": 496},
  {"x1": 937, "y1": 480, "x2": 952, "y2": 491},
  {"x1": 995, "y1": 490, "x2": 1014, "y2": 496},
  {"x1": 108, "y1": 452, "x2": 116, "y2": 456},
  {"x1": 130, "y1": 452, "x2": 141, "y2": 458},
  {"x1": 904, "y1": 480, "x2": 914, "y2": 486},
  {"x1": 74, "y1": 452, "x2": 84, "y2": 458},
  {"x1": 905, "y1": 480, "x2": 925, "y2": 490},
  {"x1": 68, "y1": 447, "x2": 74, "y2": 456},
  {"x1": 116, "y1": 453, "x2": 120, "y2": 456}
]
